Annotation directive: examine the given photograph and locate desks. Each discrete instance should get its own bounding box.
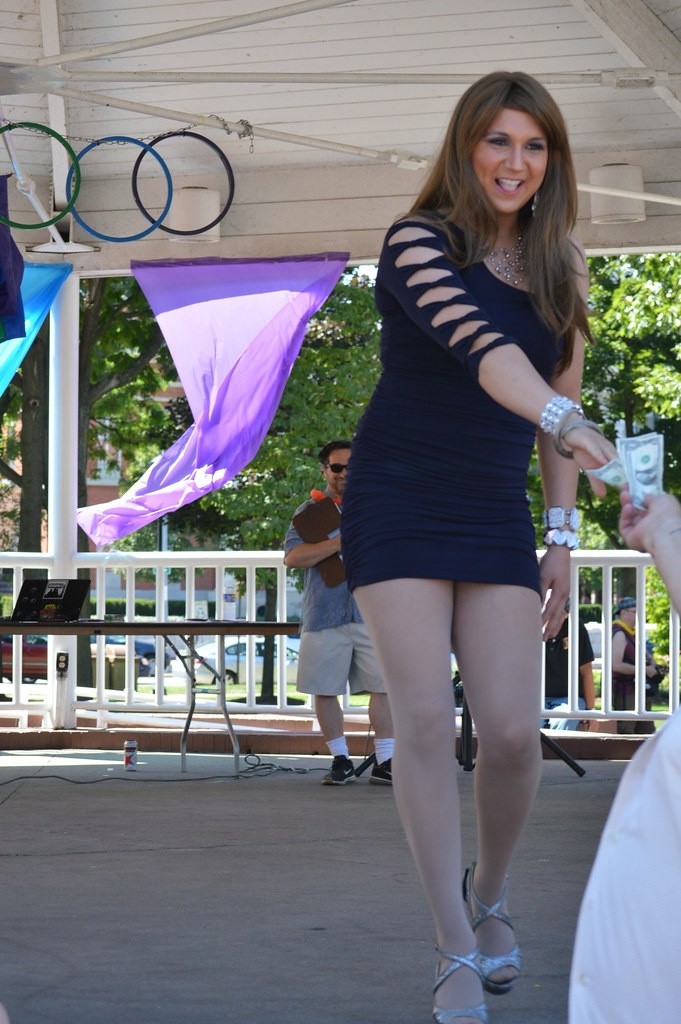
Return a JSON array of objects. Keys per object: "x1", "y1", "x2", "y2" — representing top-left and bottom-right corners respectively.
[{"x1": 0, "y1": 618, "x2": 304, "y2": 778}]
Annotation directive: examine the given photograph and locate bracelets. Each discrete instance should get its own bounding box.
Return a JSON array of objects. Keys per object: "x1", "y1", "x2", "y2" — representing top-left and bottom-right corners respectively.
[
  {"x1": 540, "y1": 394, "x2": 604, "y2": 458},
  {"x1": 651, "y1": 518, "x2": 681, "y2": 559},
  {"x1": 542, "y1": 505, "x2": 580, "y2": 550}
]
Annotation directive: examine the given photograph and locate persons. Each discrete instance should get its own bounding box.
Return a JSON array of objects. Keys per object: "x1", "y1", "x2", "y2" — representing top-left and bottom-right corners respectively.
[
  {"x1": 611, "y1": 596, "x2": 664, "y2": 734},
  {"x1": 567, "y1": 482, "x2": 681, "y2": 1024},
  {"x1": 340, "y1": 71, "x2": 630, "y2": 1024},
  {"x1": 541, "y1": 608, "x2": 599, "y2": 732},
  {"x1": 284, "y1": 441, "x2": 395, "y2": 785}
]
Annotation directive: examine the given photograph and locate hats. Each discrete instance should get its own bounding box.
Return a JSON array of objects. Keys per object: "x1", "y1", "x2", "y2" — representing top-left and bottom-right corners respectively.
[{"x1": 612, "y1": 597, "x2": 637, "y2": 615}]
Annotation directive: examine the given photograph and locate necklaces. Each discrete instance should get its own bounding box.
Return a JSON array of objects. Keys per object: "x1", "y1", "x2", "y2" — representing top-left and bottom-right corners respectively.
[{"x1": 488, "y1": 235, "x2": 528, "y2": 285}]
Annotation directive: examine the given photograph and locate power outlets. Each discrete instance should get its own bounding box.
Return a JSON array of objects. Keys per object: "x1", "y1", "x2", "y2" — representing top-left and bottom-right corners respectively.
[{"x1": 57, "y1": 652, "x2": 68, "y2": 672}]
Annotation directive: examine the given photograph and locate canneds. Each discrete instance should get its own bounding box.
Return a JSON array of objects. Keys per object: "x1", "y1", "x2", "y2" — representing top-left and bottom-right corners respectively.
[{"x1": 124, "y1": 740, "x2": 138, "y2": 771}]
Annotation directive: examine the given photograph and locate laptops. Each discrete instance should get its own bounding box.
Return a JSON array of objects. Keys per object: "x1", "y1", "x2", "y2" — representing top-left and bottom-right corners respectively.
[{"x1": 0, "y1": 579, "x2": 91, "y2": 621}]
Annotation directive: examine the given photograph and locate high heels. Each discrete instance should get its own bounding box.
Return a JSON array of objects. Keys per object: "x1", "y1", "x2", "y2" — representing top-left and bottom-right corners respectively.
[{"x1": 433, "y1": 861, "x2": 523, "y2": 1024}]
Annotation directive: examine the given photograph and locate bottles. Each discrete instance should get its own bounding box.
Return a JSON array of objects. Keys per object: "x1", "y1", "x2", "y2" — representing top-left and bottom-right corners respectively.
[{"x1": 223, "y1": 583, "x2": 235, "y2": 619}]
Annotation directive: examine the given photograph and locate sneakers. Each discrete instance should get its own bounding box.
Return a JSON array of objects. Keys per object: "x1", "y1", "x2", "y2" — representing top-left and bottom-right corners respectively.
[
  {"x1": 369, "y1": 759, "x2": 394, "y2": 786},
  {"x1": 322, "y1": 755, "x2": 357, "y2": 785}
]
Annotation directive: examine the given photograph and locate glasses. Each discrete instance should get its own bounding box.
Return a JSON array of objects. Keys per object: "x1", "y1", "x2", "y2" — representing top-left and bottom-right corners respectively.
[{"x1": 327, "y1": 462, "x2": 348, "y2": 473}]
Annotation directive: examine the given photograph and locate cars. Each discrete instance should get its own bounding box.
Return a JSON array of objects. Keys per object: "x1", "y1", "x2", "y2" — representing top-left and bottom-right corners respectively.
[
  {"x1": 170, "y1": 634, "x2": 300, "y2": 687},
  {"x1": 0, "y1": 630, "x2": 170, "y2": 687}
]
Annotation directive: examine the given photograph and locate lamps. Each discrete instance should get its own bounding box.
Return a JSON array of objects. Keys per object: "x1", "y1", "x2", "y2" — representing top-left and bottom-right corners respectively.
[
  {"x1": 590, "y1": 162, "x2": 646, "y2": 224},
  {"x1": 168, "y1": 185, "x2": 222, "y2": 245}
]
